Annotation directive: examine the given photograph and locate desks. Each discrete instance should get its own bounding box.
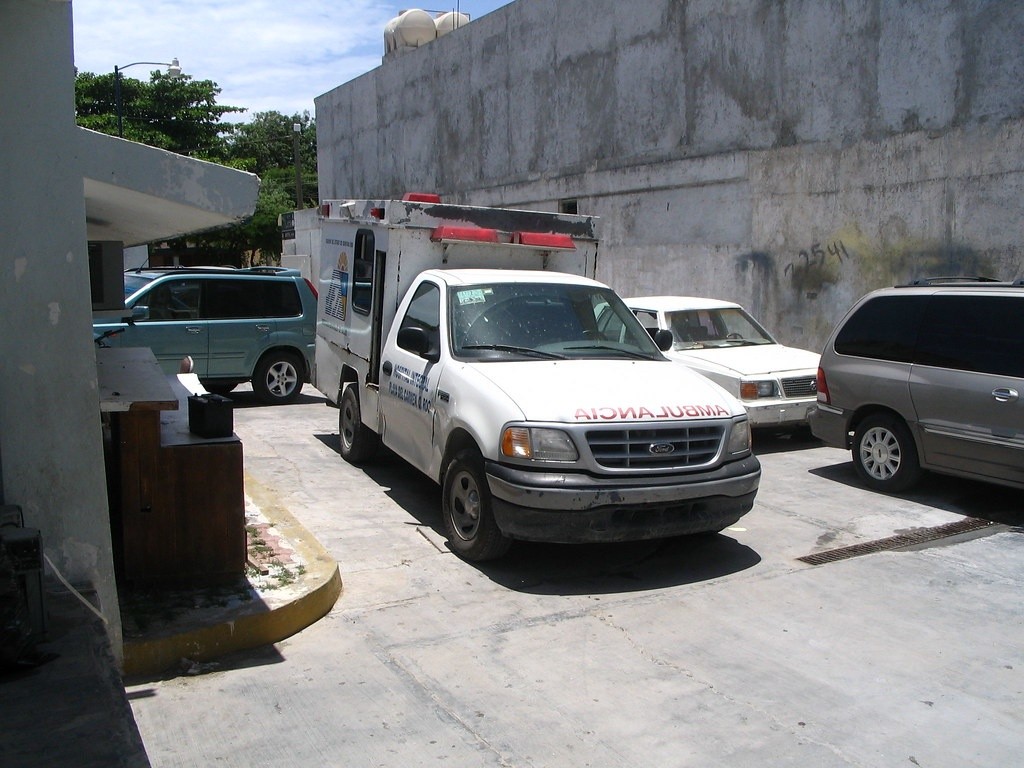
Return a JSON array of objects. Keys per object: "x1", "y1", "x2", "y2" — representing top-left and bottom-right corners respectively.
[{"x1": 96, "y1": 346, "x2": 247, "y2": 591}]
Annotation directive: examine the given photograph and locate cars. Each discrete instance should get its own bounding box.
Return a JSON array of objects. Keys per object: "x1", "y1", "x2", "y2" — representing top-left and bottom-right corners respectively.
[
  {"x1": 586, "y1": 296, "x2": 823, "y2": 439},
  {"x1": 801, "y1": 276, "x2": 1024, "y2": 493}
]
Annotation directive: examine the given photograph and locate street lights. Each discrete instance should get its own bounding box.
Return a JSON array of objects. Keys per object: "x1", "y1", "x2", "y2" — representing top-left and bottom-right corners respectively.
[{"x1": 111, "y1": 58, "x2": 183, "y2": 139}]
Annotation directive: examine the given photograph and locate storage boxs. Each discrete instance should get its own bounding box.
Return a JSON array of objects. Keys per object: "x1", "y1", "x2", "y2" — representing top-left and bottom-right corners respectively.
[{"x1": 186, "y1": 392, "x2": 234, "y2": 441}]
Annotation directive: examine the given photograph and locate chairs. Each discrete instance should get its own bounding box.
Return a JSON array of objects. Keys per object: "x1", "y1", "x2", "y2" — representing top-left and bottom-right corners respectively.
[
  {"x1": 177, "y1": 288, "x2": 199, "y2": 319},
  {"x1": 145, "y1": 285, "x2": 173, "y2": 319},
  {"x1": 180, "y1": 356, "x2": 195, "y2": 373},
  {"x1": 541, "y1": 306, "x2": 579, "y2": 344},
  {"x1": 687, "y1": 326, "x2": 708, "y2": 341}
]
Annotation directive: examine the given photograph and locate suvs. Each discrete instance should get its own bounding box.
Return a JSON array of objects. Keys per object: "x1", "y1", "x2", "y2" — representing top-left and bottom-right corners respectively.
[{"x1": 91, "y1": 263, "x2": 320, "y2": 401}]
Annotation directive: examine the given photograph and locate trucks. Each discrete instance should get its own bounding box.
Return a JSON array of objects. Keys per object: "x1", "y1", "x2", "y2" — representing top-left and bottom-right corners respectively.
[{"x1": 315, "y1": 193, "x2": 763, "y2": 562}]
